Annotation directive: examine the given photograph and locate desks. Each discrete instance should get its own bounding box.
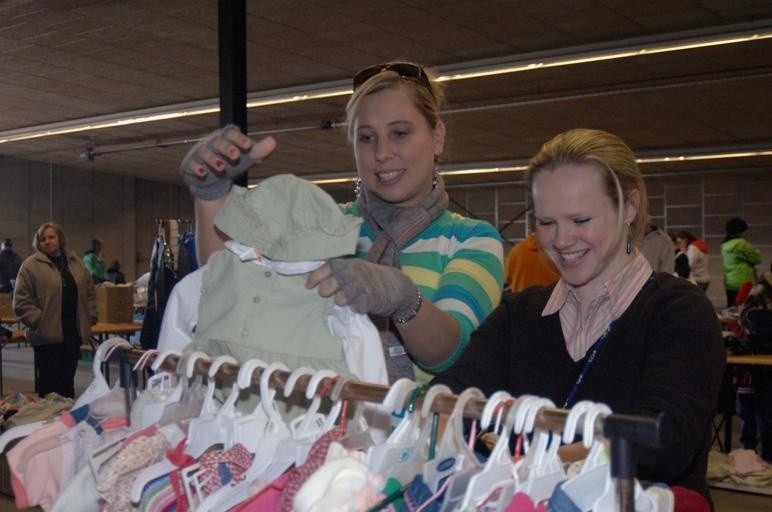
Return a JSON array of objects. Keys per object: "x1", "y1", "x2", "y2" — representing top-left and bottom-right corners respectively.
[
  {"x1": 91, "y1": 322, "x2": 142, "y2": 343},
  {"x1": 711, "y1": 354, "x2": 771, "y2": 462}
]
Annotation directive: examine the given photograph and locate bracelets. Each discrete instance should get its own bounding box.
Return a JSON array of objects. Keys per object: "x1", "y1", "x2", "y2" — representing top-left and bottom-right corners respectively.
[{"x1": 394, "y1": 285, "x2": 424, "y2": 325}]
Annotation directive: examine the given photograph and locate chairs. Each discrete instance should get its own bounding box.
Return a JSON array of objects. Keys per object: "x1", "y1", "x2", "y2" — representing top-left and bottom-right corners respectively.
[{"x1": 0, "y1": 292, "x2": 27, "y2": 348}]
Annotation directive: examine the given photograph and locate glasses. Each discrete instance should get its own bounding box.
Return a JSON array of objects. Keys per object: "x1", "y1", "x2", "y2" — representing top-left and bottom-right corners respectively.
[{"x1": 353, "y1": 64, "x2": 434, "y2": 106}]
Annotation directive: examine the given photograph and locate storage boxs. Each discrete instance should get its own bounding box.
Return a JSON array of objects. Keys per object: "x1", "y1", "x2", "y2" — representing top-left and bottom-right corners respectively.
[{"x1": 96, "y1": 286, "x2": 133, "y2": 323}]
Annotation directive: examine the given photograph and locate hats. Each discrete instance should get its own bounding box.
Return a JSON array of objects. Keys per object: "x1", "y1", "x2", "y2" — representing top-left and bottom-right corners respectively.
[{"x1": 726, "y1": 218, "x2": 748, "y2": 234}]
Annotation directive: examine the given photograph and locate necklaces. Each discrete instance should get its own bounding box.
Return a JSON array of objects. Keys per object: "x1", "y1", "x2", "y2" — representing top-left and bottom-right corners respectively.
[{"x1": 538, "y1": 315, "x2": 613, "y2": 411}]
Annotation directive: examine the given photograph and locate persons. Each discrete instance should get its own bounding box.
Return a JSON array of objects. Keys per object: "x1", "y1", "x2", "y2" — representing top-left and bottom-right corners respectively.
[
  {"x1": 505, "y1": 211, "x2": 561, "y2": 293},
  {"x1": 720, "y1": 217, "x2": 762, "y2": 307},
  {"x1": 636, "y1": 217, "x2": 711, "y2": 309},
  {"x1": 177, "y1": 59, "x2": 507, "y2": 392},
  {"x1": 423, "y1": 128, "x2": 728, "y2": 512},
  {"x1": 0, "y1": 222, "x2": 126, "y2": 399}
]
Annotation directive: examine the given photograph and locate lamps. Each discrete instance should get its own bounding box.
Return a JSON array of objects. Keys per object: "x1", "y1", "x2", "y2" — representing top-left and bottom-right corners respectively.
[{"x1": 1, "y1": 19, "x2": 771, "y2": 190}]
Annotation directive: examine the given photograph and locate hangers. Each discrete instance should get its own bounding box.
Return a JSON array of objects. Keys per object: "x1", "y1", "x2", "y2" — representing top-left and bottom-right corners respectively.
[
  {"x1": 0, "y1": 335, "x2": 657, "y2": 512},
  {"x1": 155, "y1": 218, "x2": 196, "y2": 245}
]
